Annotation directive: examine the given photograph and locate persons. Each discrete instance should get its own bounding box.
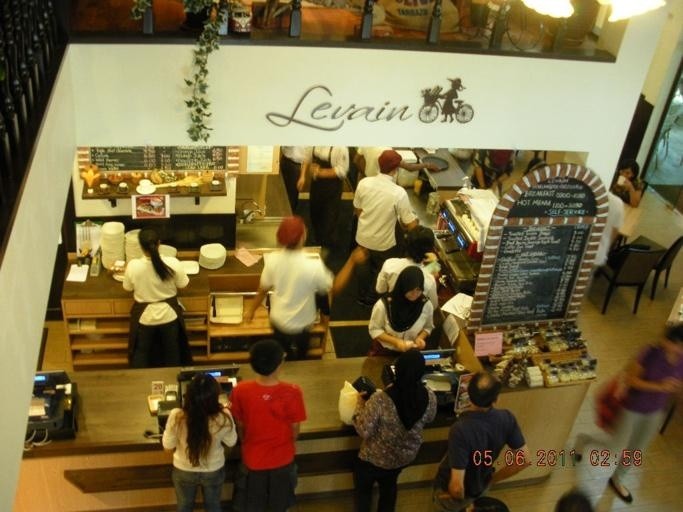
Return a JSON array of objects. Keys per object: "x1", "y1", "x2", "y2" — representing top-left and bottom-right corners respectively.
[
  {"x1": 230, "y1": 340, "x2": 307, "y2": 512},
  {"x1": 433, "y1": 369, "x2": 531, "y2": 512},
  {"x1": 280, "y1": 145, "x2": 307, "y2": 212},
  {"x1": 349, "y1": 147, "x2": 440, "y2": 253},
  {"x1": 296, "y1": 145, "x2": 349, "y2": 245},
  {"x1": 553, "y1": 488, "x2": 592, "y2": 512},
  {"x1": 369, "y1": 267, "x2": 436, "y2": 355},
  {"x1": 609, "y1": 160, "x2": 645, "y2": 209},
  {"x1": 577, "y1": 322, "x2": 683, "y2": 508},
  {"x1": 351, "y1": 348, "x2": 437, "y2": 512},
  {"x1": 471, "y1": 150, "x2": 515, "y2": 190},
  {"x1": 353, "y1": 150, "x2": 418, "y2": 308},
  {"x1": 375, "y1": 225, "x2": 439, "y2": 311},
  {"x1": 123, "y1": 229, "x2": 190, "y2": 370},
  {"x1": 162, "y1": 373, "x2": 237, "y2": 512},
  {"x1": 242, "y1": 216, "x2": 367, "y2": 361}
]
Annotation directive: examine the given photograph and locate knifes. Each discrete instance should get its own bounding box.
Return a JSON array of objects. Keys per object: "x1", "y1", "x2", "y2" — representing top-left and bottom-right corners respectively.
[{"x1": 210, "y1": 295, "x2": 216, "y2": 317}]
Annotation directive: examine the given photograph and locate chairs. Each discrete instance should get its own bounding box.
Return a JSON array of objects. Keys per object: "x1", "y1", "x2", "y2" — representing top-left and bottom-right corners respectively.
[
  {"x1": 627, "y1": 234, "x2": 683, "y2": 300},
  {"x1": 593, "y1": 244, "x2": 664, "y2": 317}
]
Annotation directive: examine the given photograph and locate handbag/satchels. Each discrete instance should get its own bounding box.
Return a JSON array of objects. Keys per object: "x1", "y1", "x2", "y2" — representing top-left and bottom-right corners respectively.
[{"x1": 596, "y1": 375, "x2": 632, "y2": 429}]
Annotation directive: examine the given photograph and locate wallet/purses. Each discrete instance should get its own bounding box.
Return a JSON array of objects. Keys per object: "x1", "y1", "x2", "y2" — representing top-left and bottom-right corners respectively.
[{"x1": 352, "y1": 376, "x2": 376, "y2": 401}]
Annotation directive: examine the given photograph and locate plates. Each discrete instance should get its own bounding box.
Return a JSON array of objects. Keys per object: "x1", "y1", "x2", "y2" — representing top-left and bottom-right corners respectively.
[
  {"x1": 617, "y1": 175, "x2": 626, "y2": 186},
  {"x1": 135, "y1": 186, "x2": 156, "y2": 194},
  {"x1": 100, "y1": 221, "x2": 124, "y2": 271},
  {"x1": 198, "y1": 243, "x2": 227, "y2": 270},
  {"x1": 178, "y1": 260, "x2": 198, "y2": 275},
  {"x1": 420, "y1": 155, "x2": 450, "y2": 170},
  {"x1": 124, "y1": 229, "x2": 142, "y2": 262},
  {"x1": 158, "y1": 244, "x2": 176, "y2": 259}
]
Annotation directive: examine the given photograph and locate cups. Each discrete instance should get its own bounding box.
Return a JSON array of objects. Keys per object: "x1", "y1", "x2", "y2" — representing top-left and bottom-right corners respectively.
[
  {"x1": 210, "y1": 180, "x2": 220, "y2": 190},
  {"x1": 188, "y1": 182, "x2": 199, "y2": 192},
  {"x1": 138, "y1": 179, "x2": 151, "y2": 191},
  {"x1": 118, "y1": 183, "x2": 129, "y2": 193},
  {"x1": 99, "y1": 184, "x2": 108, "y2": 194}
]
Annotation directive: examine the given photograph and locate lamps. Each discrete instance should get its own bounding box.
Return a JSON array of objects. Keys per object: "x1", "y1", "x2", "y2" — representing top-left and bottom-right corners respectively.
[
  {"x1": 608, "y1": 0, "x2": 666, "y2": 23},
  {"x1": 521, "y1": 0, "x2": 574, "y2": 18}
]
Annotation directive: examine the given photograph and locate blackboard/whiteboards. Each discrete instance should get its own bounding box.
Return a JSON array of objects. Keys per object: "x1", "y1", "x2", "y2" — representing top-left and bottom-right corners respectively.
[
  {"x1": 504, "y1": 176, "x2": 600, "y2": 220},
  {"x1": 481, "y1": 225, "x2": 592, "y2": 324},
  {"x1": 90, "y1": 146, "x2": 227, "y2": 172}
]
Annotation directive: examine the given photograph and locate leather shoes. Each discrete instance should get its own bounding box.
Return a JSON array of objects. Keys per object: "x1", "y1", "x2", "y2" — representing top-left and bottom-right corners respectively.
[{"x1": 610, "y1": 477, "x2": 633, "y2": 503}]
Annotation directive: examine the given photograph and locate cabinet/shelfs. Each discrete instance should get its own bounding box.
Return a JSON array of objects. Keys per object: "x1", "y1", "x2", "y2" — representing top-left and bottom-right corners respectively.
[
  {"x1": 60, "y1": 247, "x2": 334, "y2": 372},
  {"x1": 455, "y1": 328, "x2": 598, "y2": 491}
]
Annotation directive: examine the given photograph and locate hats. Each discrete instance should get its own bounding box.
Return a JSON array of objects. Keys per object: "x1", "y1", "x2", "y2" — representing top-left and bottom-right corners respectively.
[
  {"x1": 277, "y1": 217, "x2": 304, "y2": 245},
  {"x1": 379, "y1": 151, "x2": 402, "y2": 174}
]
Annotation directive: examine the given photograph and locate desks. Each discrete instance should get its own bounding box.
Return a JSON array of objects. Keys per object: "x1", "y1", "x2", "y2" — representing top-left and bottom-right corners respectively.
[{"x1": 10, "y1": 355, "x2": 459, "y2": 512}]
